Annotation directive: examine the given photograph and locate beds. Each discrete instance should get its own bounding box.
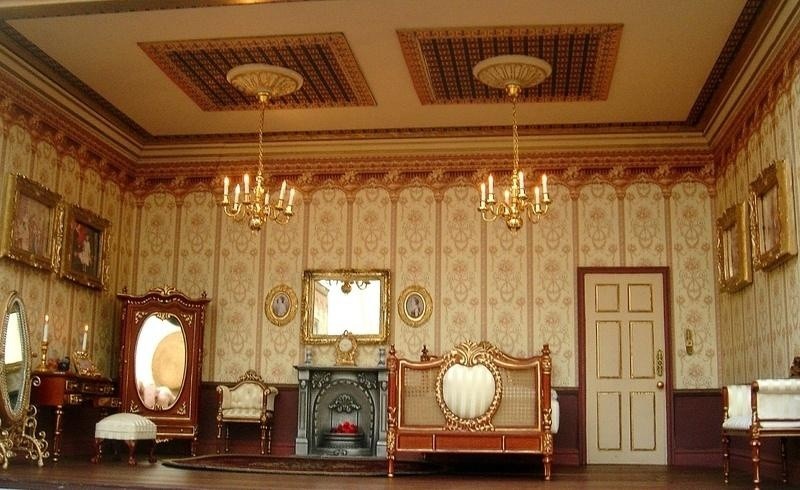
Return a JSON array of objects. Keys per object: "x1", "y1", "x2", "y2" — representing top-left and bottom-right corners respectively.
[{"x1": 386, "y1": 334, "x2": 561, "y2": 481}]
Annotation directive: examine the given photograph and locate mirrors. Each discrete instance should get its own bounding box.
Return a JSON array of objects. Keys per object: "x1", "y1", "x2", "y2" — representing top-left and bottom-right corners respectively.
[
  {"x1": 133, "y1": 311, "x2": 189, "y2": 412},
  {"x1": 0, "y1": 289, "x2": 50, "y2": 470},
  {"x1": 299, "y1": 268, "x2": 392, "y2": 343}
]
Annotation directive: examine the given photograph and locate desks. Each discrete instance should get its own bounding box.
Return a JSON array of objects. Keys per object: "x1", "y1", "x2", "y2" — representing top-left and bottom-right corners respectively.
[{"x1": 31, "y1": 368, "x2": 120, "y2": 462}]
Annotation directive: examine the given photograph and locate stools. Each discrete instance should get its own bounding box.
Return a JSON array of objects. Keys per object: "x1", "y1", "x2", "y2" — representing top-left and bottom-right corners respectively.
[{"x1": 90, "y1": 410, "x2": 158, "y2": 466}]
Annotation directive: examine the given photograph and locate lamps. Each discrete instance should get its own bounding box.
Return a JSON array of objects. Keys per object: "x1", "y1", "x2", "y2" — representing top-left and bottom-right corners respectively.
[
  {"x1": 221, "y1": 62, "x2": 302, "y2": 231},
  {"x1": 473, "y1": 55, "x2": 554, "y2": 238}
]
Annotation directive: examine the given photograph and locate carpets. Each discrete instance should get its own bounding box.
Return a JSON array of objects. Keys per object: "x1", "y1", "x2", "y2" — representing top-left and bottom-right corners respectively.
[{"x1": 161, "y1": 453, "x2": 460, "y2": 478}]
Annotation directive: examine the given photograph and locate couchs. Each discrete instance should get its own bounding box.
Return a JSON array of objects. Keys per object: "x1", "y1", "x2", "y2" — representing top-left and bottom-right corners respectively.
[
  {"x1": 213, "y1": 368, "x2": 279, "y2": 454},
  {"x1": 721, "y1": 354, "x2": 800, "y2": 490}
]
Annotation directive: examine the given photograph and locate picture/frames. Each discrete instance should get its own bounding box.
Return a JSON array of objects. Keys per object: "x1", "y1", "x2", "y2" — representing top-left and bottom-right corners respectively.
[
  {"x1": 59, "y1": 202, "x2": 113, "y2": 293},
  {"x1": 746, "y1": 157, "x2": 798, "y2": 271},
  {"x1": 713, "y1": 199, "x2": 753, "y2": 295},
  {"x1": 397, "y1": 284, "x2": 433, "y2": 328},
  {"x1": 263, "y1": 283, "x2": 297, "y2": 328},
  {"x1": 1, "y1": 170, "x2": 65, "y2": 274}
]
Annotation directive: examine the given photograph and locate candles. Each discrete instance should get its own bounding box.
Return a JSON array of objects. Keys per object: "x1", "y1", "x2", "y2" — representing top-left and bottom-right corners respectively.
[
  {"x1": 82, "y1": 324, "x2": 89, "y2": 351},
  {"x1": 42, "y1": 314, "x2": 49, "y2": 341}
]
